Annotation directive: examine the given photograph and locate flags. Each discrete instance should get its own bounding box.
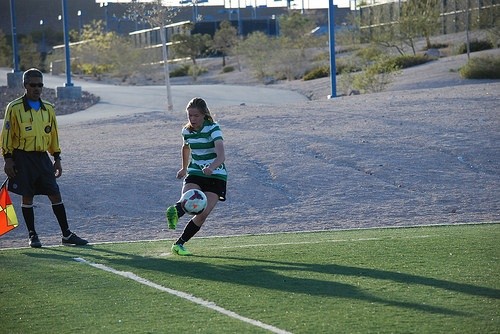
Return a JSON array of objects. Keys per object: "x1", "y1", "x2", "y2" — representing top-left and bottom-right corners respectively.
[{"x1": 0, "y1": 185, "x2": 19, "y2": 236}]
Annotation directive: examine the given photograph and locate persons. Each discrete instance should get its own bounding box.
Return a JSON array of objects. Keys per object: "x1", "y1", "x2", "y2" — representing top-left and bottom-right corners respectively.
[
  {"x1": 166, "y1": 96, "x2": 227, "y2": 256},
  {"x1": 0, "y1": 68, "x2": 89, "y2": 247}
]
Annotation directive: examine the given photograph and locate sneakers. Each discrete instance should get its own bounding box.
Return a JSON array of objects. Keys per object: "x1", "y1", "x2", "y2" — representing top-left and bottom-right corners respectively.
[
  {"x1": 166, "y1": 205, "x2": 178, "y2": 230},
  {"x1": 61, "y1": 232, "x2": 89, "y2": 245},
  {"x1": 28, "y1": 235, "x2": 43, "y2": 248},
  {"x1": 170, "y1": 243, "x2": 192, "y2": 256}
]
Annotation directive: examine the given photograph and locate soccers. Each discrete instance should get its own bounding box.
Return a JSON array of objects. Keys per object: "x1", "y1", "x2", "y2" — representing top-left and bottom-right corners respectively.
[{"x1": 180, "y1": 188, "x2": 207, "y2": 215}]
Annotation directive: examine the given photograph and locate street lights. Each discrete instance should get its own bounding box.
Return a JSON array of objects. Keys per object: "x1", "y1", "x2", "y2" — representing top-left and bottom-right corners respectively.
[{"x1": 179, "y1": 0, "x2": 209, "y2": 25}]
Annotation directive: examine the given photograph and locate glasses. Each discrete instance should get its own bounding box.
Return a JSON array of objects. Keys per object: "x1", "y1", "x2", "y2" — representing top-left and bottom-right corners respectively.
[{"x1": 24, "y1": 82, "x2": 44, "y2": 88}]
